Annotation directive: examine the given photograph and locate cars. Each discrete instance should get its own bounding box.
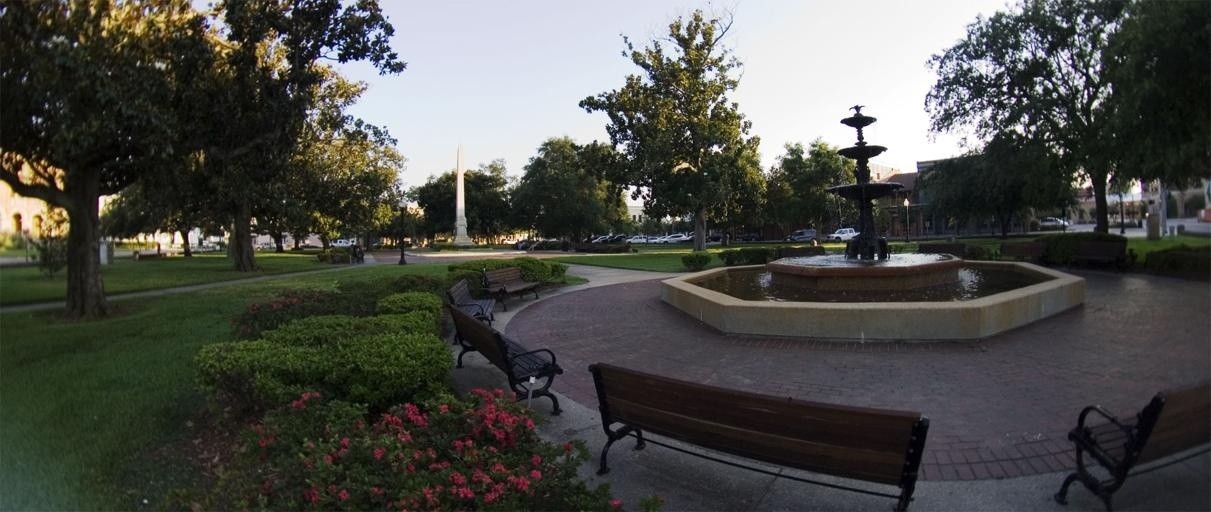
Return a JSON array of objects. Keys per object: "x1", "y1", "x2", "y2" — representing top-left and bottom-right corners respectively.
[
  {"x1": 582, "y1": 232, "x2": 760, "y2": 245},
  {"x1": 1038, "y1": 216, "x2": 1070, "y2": 230}
]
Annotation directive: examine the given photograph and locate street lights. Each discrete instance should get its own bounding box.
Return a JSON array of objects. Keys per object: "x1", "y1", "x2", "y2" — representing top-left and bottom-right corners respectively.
[
  {"x1": 398, "y1": 201, "x2": 408, "y2": 265},
  {"x1": 903, "y1": 197, "x2": 910, "y2": 242}
]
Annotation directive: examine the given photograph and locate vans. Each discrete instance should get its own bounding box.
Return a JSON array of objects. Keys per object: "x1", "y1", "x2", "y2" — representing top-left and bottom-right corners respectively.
[{"x1": 790, "y1": 228, "x2": 818, "y2": 243}]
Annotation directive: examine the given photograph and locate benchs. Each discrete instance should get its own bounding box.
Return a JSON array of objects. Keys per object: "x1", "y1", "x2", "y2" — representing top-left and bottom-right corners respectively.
[{"x1": 1054, "y1": 384, "x2": 1211, "y2": 511}]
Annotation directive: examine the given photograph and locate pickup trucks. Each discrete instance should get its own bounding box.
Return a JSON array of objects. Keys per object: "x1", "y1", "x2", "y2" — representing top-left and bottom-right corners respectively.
[{"x1": 826, "y1": 227, "x2": 861, "y2": 243}]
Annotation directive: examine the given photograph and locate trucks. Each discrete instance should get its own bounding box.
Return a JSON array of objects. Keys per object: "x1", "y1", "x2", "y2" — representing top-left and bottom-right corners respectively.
[{"x1": 329, "y1": 239, "x2": 350, "y2": 246}]
